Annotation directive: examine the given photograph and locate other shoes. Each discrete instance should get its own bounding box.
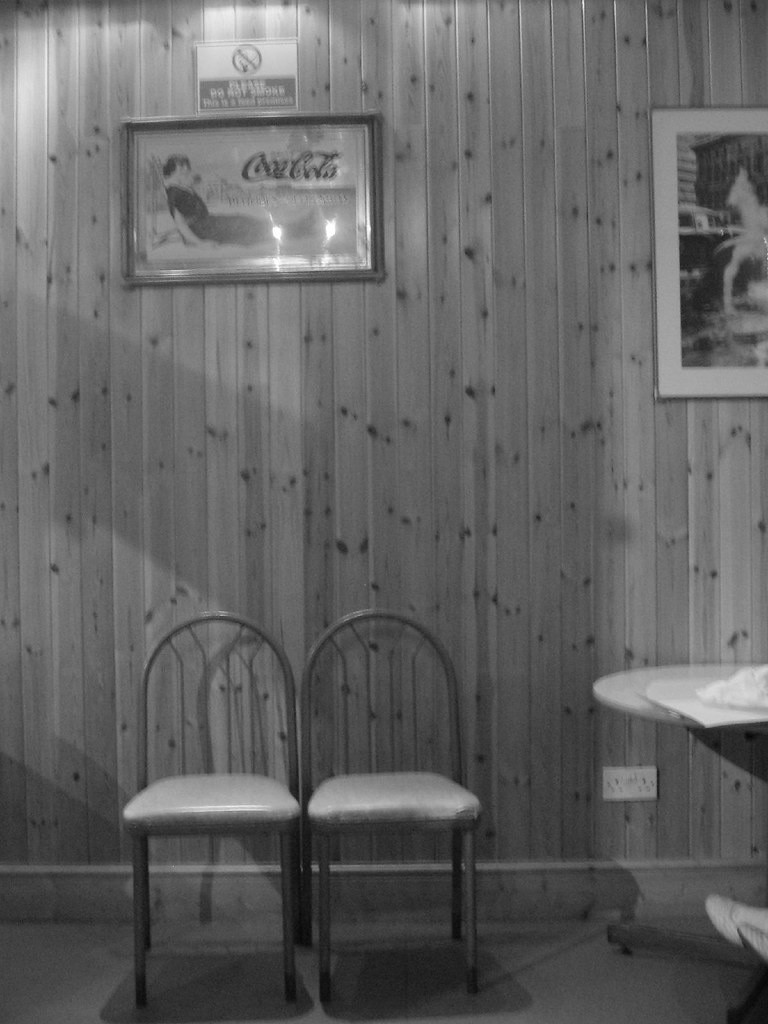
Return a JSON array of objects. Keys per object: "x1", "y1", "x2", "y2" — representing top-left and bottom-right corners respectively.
[{"x1": 704, "y1": 895, "x2": 768, "y2": 959}]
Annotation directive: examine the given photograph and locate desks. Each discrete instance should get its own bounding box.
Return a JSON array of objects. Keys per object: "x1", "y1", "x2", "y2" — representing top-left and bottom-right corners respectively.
[{"x1": 591, "y1": 663, "x2": 768, "y2": 1024}]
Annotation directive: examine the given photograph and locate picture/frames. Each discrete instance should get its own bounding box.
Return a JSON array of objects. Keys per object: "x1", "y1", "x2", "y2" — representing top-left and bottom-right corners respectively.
[
  {"x1": 118, "y1": 111, "x2": 388, "y2": 285},
  {"x1": 650, "y1": 106, "x2": 768, "y2": 400}
]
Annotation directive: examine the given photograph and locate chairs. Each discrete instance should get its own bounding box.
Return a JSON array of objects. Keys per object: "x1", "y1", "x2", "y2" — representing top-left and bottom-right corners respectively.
[
  {"x1": 298, "y1": 606, "x2": 479, "y2": 1006},
  {"x1": 123, "y1": 612, "x2": 307, "y2": 1006}
]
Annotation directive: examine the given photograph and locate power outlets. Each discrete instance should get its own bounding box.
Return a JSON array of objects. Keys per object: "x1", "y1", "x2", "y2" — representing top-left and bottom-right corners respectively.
[{"x1": 603, "y1": 767, "x2": 657, "y2": 801}]
